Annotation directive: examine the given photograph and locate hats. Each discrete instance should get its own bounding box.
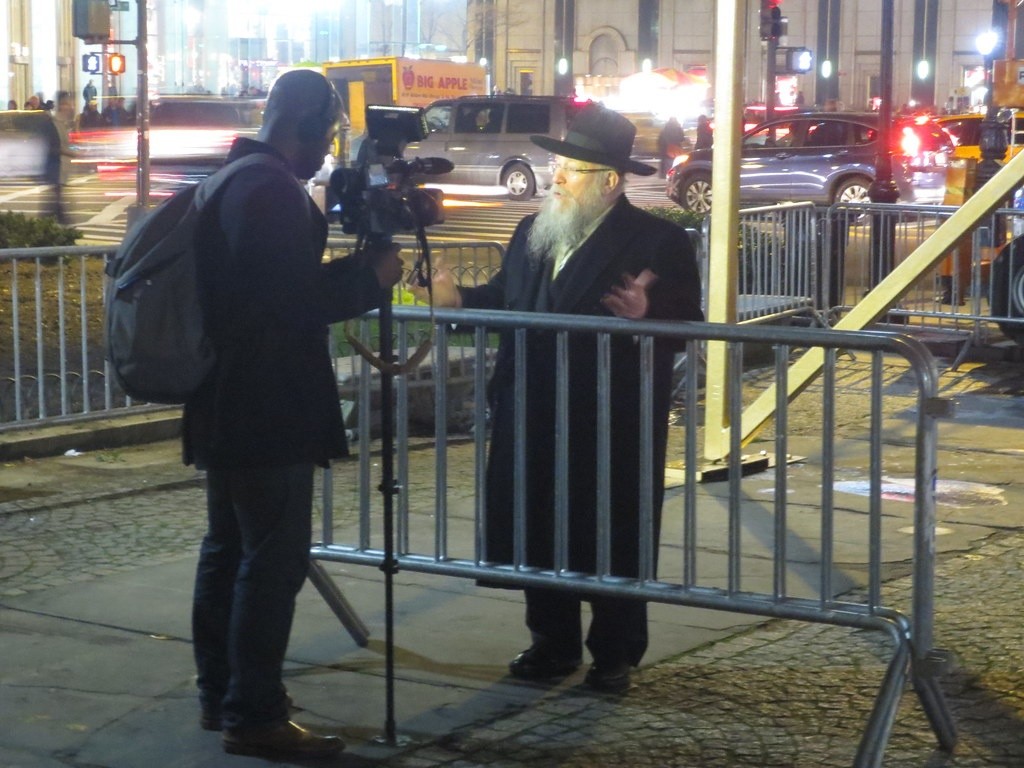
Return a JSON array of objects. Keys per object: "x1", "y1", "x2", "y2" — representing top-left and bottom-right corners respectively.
[{"x1": 530, "y1": 104, "x2": 659, "y2": 178}]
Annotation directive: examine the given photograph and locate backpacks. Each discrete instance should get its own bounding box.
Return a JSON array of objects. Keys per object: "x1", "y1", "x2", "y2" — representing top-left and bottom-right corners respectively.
[{"x1": 98, "y1": 149, "x2": 310, "y2": 404}]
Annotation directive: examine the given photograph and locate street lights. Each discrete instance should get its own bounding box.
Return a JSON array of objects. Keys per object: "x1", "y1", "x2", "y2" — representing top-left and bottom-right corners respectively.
[{"x1": 978, "y1": 32, "x2": 997, "y2": 87}]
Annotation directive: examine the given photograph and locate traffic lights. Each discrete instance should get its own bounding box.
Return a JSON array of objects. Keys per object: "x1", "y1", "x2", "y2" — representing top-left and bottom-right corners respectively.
[
  {"x1": 787, "y1": 50, "x2": 812, "y2": 73},
  {"x1": 83, "y1": 54, "x2": 99, "y2": 72},
  {"x1": 109, "y1": 56, "x2": 125, "y2": 73},
  {"x1": 760, "y1": 0, "x2": 781, "y2": 38}
]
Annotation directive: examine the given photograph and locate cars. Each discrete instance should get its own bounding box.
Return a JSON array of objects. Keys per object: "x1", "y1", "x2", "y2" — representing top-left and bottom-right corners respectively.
[
  {"x1": 0, "y1": 96, "x2": 267, "y2": 178},
  {"x1": 934, "y1": 113, "x2": 1024, "y2": 164},
  {"x1": 666, "y1": 113, "x2": 955, "y2": 226},
  {"x1": 621, "y1": 107, "x2": 817, "y2": 155}
]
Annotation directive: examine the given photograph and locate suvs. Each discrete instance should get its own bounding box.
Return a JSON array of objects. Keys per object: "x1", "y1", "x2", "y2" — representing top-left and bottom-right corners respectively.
[{"x1": 402, "y1": 95, "x2": 572, "y2": 202}]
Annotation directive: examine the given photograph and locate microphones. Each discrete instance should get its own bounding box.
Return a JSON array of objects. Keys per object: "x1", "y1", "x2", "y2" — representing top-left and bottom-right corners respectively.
[{"x1": 413, "y1": 157, "x2": 454, "y2": 174}]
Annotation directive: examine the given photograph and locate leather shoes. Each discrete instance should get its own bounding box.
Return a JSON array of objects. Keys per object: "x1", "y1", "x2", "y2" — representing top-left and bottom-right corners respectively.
[
  {"x1": 199, "y1": 710, "x2": 229, "y2": 731},
  {"x1": 507, "y1": 638, "x2": 583, "y2": 679},
  {"x1": 585, "y1": 658, "x2": 632, "y2": 690},
  {"x1": 221, "y1": 717, "x2": 346, "y2": 764}
]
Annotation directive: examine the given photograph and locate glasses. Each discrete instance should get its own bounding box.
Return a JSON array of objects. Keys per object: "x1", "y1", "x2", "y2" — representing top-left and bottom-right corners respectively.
[{"x1": 548, "y1": 162, "x2": 612, "y2": 184}]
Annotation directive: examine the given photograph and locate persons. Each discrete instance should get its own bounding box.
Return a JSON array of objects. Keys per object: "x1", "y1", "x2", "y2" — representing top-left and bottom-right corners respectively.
[
  {"x1": 817, "y1": 100, "x2": 842, "y2": 144},
  {"x1": 796, "y1": 92, "x2": 804, "y2": 104},
  {"x1": 181, "y1": 68, "x2": 404, "y2": 758},
  {"x1": 770, "y1": 123, "x2": 797, "y2": 147},
  {"x1": 7, "y1": 91, "x2": 84, "y2": 223},
  {"x1": 658, "y1": 115, "x2": 713, "y2": 179},
  {"x1": 81, "y1": 79, "x2": 130, "y2": 126},
  {"x1": 408, "y1": 107, "x2": 707, "y2": 687}
]
